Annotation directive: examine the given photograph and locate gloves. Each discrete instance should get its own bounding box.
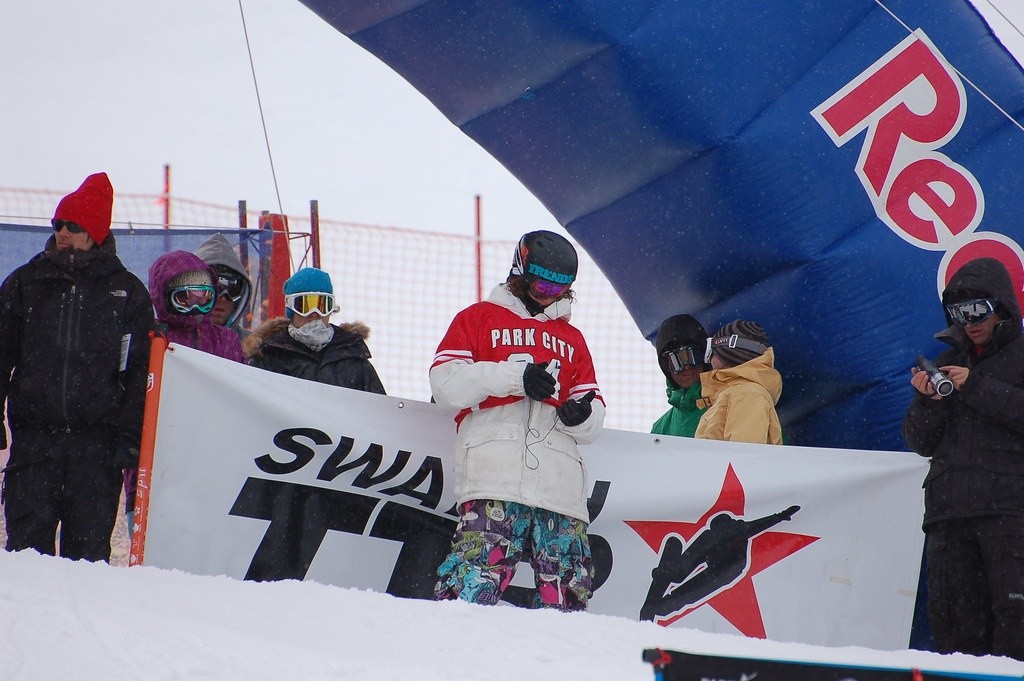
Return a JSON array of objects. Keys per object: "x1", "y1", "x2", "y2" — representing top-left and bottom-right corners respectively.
[
  {"x1": 523, "y1": 361, "x2": 557, "y2": 400},
  {"x1": 102, "y1": 437, "x2": 140, "y2": 467},
  {"x1": 557, "y1": 390, "x2": 597, "y2": 426}
]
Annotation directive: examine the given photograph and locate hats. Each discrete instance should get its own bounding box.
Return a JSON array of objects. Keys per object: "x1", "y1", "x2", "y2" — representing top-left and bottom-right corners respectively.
[
  {"x1": 283, "y1": 268, "x2": 334, "y2": 318},
  {"x1": 712, "y1": 319, "x2": 769, "y2": 367},
  {"x1": 54, "y1": 172, "x2": 113, "y2": 247},
  {"x1": 657, "y1": 314, "x2": 710, "y2": 389},
  {"x1": 164, "y1": 270, "x2": 212, "y2": 294}
]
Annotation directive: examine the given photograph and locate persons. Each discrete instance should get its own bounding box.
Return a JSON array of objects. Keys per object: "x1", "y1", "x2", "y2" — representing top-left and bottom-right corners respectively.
[
  {"x1": 195, "y1": 232, "x2": 253, "y2": 340},
  {"x1": 243, "y1": 267, "x2": 386, "y2": 395},
  {"x1": 429, "y1": 229, "x2": 606, "y2": 614},
  {"x1": 695, "y1": 319, "x2": 784, "y2": 445},
  {"x1": 904, "y1": 257, "x2": 1024, "y2": 660},
  {"x1": 0, "y1": 172, "x2": 154, "y2": 565},
  {"x1": 124, "y1": 250, "x2": 244, "y2": 540},
  {"x1": 650, "y1": 315, "x2": 788, "y2": 444}
]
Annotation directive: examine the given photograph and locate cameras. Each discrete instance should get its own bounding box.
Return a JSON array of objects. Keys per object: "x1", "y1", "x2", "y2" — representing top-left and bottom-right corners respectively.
[{"x1": 915, "y1": 356, "x2": 954, "y2": 397}]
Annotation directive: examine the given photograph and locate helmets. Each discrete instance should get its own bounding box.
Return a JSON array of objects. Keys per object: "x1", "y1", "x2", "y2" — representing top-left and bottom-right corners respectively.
[{"x1": 512, "y1": 229, "x2": 578, "y2": 287}]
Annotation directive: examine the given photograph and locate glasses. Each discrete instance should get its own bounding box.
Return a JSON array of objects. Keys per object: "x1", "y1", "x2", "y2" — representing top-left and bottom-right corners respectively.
[
  {"x1": 286, "y1": 292, "x2": 337, "y2": 317},
  {"x1": 527, "y1": 277, "x2": 570, "y2": 300},
  {"x1": 216, "y1": 274, "x2": 246, "y2": 303},
  {"x1": 52, "y1": 219, "x2": 85, "y2": 234},
  {"x1": 660, "y1": 344, "x2": 705, "y2": 374},
  {"x1": 945, "y1": 298, "x2": 994, "y2": 326},
  {"x1": 171, "y1": 285, "x2": 216, "y2": 313}
]
